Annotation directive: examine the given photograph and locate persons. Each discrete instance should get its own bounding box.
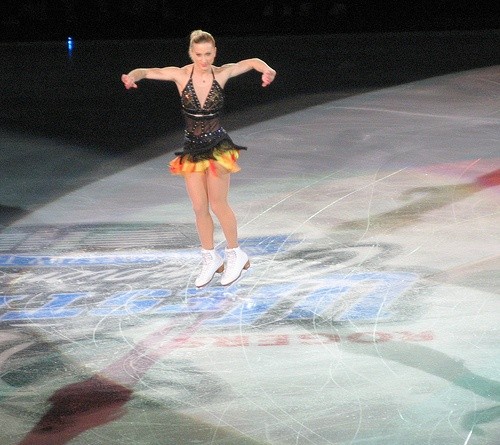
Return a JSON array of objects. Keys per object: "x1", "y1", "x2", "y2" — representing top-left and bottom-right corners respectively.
[{"x1": 122, "y1": 30, "x2": 276, "y2": 287}]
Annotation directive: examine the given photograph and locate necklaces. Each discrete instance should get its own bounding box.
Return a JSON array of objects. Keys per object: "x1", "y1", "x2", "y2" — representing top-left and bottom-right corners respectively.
[{"x1": 202, "y1": 80, "x2": 206, "y2": 83}]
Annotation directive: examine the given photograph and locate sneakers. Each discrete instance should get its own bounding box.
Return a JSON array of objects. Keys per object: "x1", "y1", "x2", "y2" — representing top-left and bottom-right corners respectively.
[
  {"x1": 221, "y1": 246, "x2": 250, "y2": 287},
  {"x1": 194, "y1": 247, "x2": 224, "y2": 289}
]
